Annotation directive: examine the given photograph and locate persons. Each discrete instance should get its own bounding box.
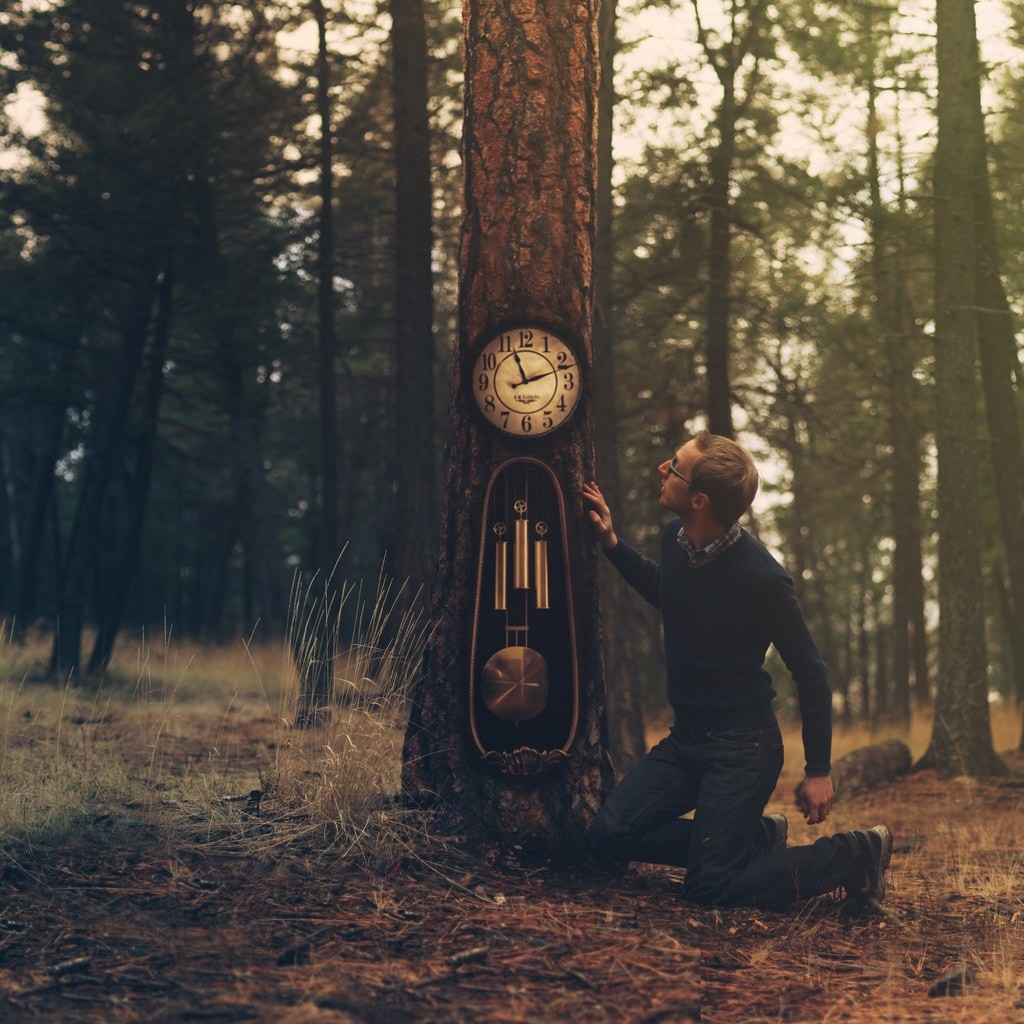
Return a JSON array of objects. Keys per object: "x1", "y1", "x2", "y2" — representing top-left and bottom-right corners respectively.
[{"x1": 579, "y1": 431, "x2": 891, "y2": 917}]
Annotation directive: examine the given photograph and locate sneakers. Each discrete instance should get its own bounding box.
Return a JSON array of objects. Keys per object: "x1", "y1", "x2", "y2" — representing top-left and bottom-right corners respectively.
[
  {"x1": 767, "y1": 814, "x2": 787, "y2": 854},
  {"x1": 834, "y1": 824, "x2": 894, "y2": 909}
]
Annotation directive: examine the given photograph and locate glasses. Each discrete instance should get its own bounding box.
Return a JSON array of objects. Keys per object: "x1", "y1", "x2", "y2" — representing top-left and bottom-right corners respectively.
[{"x1": 667, "y1": 457, "x2": 697, "y2": 490}]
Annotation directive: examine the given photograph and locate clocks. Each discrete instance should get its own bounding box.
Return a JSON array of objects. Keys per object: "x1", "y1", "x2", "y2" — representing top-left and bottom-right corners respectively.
[{"x1": 460, "y1": 318, "x2": 588, "y2": 447}]
